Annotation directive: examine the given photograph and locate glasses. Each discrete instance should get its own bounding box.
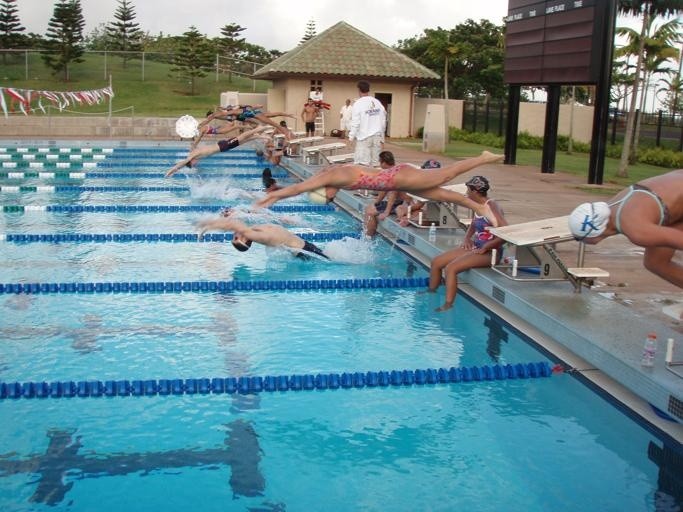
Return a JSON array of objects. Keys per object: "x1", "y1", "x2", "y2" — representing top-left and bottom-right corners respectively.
[
  {"x1": 234, "y1": 236, "x2": 249, "y2": 249},
  {"x1": 573, "y1": 202, "x2": 595, "y2": 242}
]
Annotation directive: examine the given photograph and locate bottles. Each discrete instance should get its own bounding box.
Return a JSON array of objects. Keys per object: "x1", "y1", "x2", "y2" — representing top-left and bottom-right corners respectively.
[
  {"x1": 642, "y1": 333, "x2": 658, "y2": 367},
  {"x1": 429, "y1": 223, "x2": 436, "y2": 241}
]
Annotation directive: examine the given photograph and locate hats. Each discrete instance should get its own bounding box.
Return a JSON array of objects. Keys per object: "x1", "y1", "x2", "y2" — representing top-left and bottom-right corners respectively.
[
  {"x1": 466, "y1": 176, "x2": 489, "y2": 192},
  {"x1": 569, "y1": 201, "x2": 610, "y2": 238},
  {"x1": 232, "y1": 238, "x2": 253, "y2": 252},
  {"x1": 421, "y1": 159, "x2": 440, "y2": 170}
]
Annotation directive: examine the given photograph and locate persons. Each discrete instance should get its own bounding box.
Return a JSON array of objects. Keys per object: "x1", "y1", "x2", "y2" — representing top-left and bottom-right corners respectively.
[
  {"x1": 165, "y1": 123, "x2": 274, "y2": 179},
  {"x1": 362, "y1": 151, "x2": 404, "y2": 240},
  {"x1": 417, "y1": 176, "x2": 510, "y2": 313},
  {"x1": 263, "y1": 141, "x2": 281, "y2": 165},
  {"x1": 255, "y1": 149, "x2": 282, "y2": 166},
  {"x1": 344, "y1": 81, "x2": 387, "y2": 199},
  {"x1": 301, "y1": 97, "x2": 318, "y2": 138},
  {"x1": 188, "y1": 123, "x2": 254, "y2": 151},
  {"x1": 265, "y1": 119, "x2": 295, "y2": 149},
  {"x1": 309, "y1": 86, "x2": 323, "y2": 109},
  {"x1": 338, "y1": 99, "x2": 354, "y2": 139},
  {"x1": 253, "y1": 149, "x2": 507, "y2": 229},
  {"x1": 395, "y1": 161, "x2": 445, "y2": 227},
  {"x1": 196, "y1": 109, "x2": 299, "y2": 137},
  {"x1": 567, "y1": 167, "x2": 683, "y2": 289},
  {"x1": 262, "y1": 168, "x2": 285, "y2": 194},
  {"x1": 215, "y1": 104, "x2": 266, "y2": 112},
  {"x1": 255, "y1": 149, "x2": 266, "y2": 161},
  {"x1": 195, "y1": 217, "x2": 328, "y2": 261}
]
